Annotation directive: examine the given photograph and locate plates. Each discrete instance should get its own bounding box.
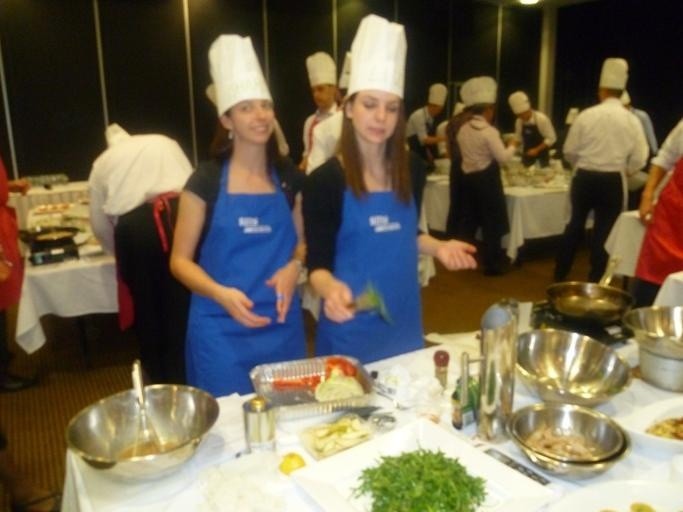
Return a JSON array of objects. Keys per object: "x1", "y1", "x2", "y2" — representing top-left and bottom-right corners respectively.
[
  {"x1": 554, "y1": 479, "x2": 683, "y2": 511},
  {"x1": 294, "y1": 416, "x2": 558, "y2": 511},
  {"x1": 612, "y1": 396, "x2": 683, "y2": 452}
]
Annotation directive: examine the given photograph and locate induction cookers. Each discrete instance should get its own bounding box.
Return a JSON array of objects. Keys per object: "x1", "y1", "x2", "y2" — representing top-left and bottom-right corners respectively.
[
  {"x1": 530, "y1": 300, "x2": 635, "y2": 348},
  {"x1": 29, "y1": 239, "x2": 79, "y2": 266}
]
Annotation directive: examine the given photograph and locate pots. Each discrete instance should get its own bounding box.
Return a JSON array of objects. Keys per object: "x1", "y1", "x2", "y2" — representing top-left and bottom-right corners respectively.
[
  {"x1": 18, "y1": 226, "x2": 79, "y2": 246},
  {"x1": 544, "y1": 254, "x2": 635, "y2": 323}
]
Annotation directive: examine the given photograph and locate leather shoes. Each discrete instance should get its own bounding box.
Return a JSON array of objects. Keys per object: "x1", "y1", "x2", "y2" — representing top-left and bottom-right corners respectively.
[{"x1": 0, "y1": 372, "x2": 42, "y2": 393}]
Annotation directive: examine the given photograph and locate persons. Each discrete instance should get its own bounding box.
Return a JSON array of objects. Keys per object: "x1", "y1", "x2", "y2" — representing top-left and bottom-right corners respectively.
[
  {"x1": 85, "y1": 123, "x2": 194, "y2": 382},
  {"x1": 169, "y1": 35, "x2": 307, "y2": 399},
  {"x1": 552, "y1": 57, "x2": 658, "y2": 284},
  {"x1": 623, "y1": 120, "x2": 682, "y2": 337},
  {"x1": 405, "y1": 84, "x2": 448, "y2": 219},
  {"x1": 305, "y1": 50, "x2": 351, "y2": 175},
  {"x1": 508, "y1": 91, "x2": 557, "y2": 167},
  {"x1": 436, "y1": 76, "x2": 520, "y2": 277},
  {"x1": 1, "y1": 156, "x2": 30, "y2": 393},
  {"x1": 297, "y1": 52, "x2": 337, "y2": 172},
  {"x1": 302, "y1": 14, "x2": 479, "y2": 364}
]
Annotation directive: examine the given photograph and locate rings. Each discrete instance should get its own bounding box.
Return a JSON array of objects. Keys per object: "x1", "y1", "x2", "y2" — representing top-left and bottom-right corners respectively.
[{"x1": 276, "y1": 295, "x2": 284, "y2": 300}]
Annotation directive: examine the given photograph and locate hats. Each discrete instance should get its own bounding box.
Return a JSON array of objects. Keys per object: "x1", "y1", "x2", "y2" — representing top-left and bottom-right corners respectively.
[
  {"x1": 207, "y1": 32, "x2": 275, "y2": 117},
  {"x1": 104, "y1": 121, "x2": 130, "y2": 149},
  {"x1": 620, "y1": 90, "x2": 633, "y2": 108},
  {"x1": 340, "y1": 51, "x2": 352, "y2": 90},
  {"x1": 598, "y1": 56, "x2": 629, "y2": 92},
  {"x1": 427, "y1": 82, "x2": 449, "y2": 108},
  {"x1": 340, "y1": 13, "x2": 407, "y2": 102},
  {"x1": 508, "y1": 89, "x2": 532, "y2": 117},
  {"x1": 453, "y1": 101, "x2": 465, "y2": 118},
  {"x1": 304, "y1": 50, "x2": 339, "y2": 87},
  {"x1": 460, "y1": 75, "x2": 498, "y2": 109}
]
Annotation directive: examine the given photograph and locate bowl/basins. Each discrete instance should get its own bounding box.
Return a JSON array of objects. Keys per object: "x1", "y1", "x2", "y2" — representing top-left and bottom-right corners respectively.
[
  {"x1": 639, "y1": 347, "x2": 683, "y2": 392},
  {"x1": 512, "y1": 328, "x2": 633, "y2": 407},
  {"x1": 505, "y1": 403, "x2": 630, "y2": 481},
  {"x1": 65, "y1": 383, "x2": 220, "y2": 486},
  {"x1": 622, "y1": 305, "x2": 683, "y2": 357}
]
